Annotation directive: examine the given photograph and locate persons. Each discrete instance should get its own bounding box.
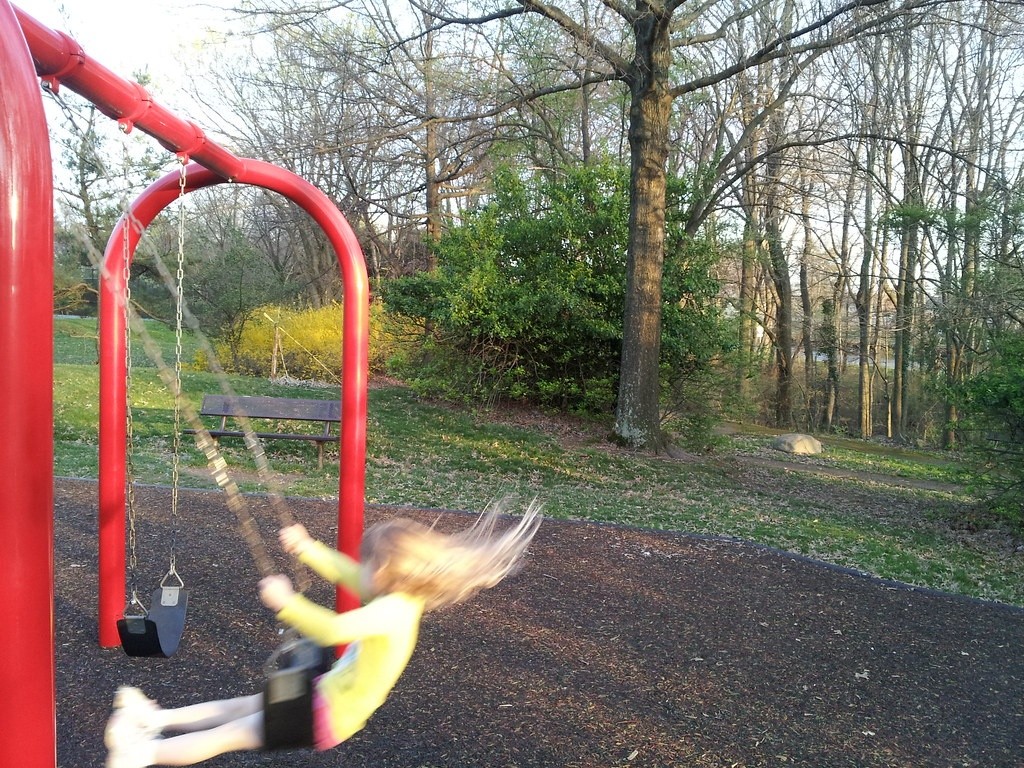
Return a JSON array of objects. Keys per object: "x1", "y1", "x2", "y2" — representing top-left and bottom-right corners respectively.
[{"x1": 104, "y1": 489, "x2": 543, "y2": 766}]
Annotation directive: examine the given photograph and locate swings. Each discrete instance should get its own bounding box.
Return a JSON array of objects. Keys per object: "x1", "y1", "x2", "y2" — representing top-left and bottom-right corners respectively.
[
  {"x1": 114, "y1": 119, "x2": 193, "y2": 661},
  {"x1": 40, "y1": 69, "x2": 330, "y2": 752}
]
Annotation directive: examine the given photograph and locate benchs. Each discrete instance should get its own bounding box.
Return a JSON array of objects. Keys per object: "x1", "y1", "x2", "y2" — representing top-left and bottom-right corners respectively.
[{"x1": 183, "y1": 395, "x2": 341, "y2": 470}]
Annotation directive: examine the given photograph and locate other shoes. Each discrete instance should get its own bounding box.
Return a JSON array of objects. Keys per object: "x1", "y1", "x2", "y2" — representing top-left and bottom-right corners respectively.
[
  {"x1": 112, "y1": 687, "x2": 166, "y2": 739},
  {"x1": 104, "y1": 709, "x2": 149, "y2": 768}
]
